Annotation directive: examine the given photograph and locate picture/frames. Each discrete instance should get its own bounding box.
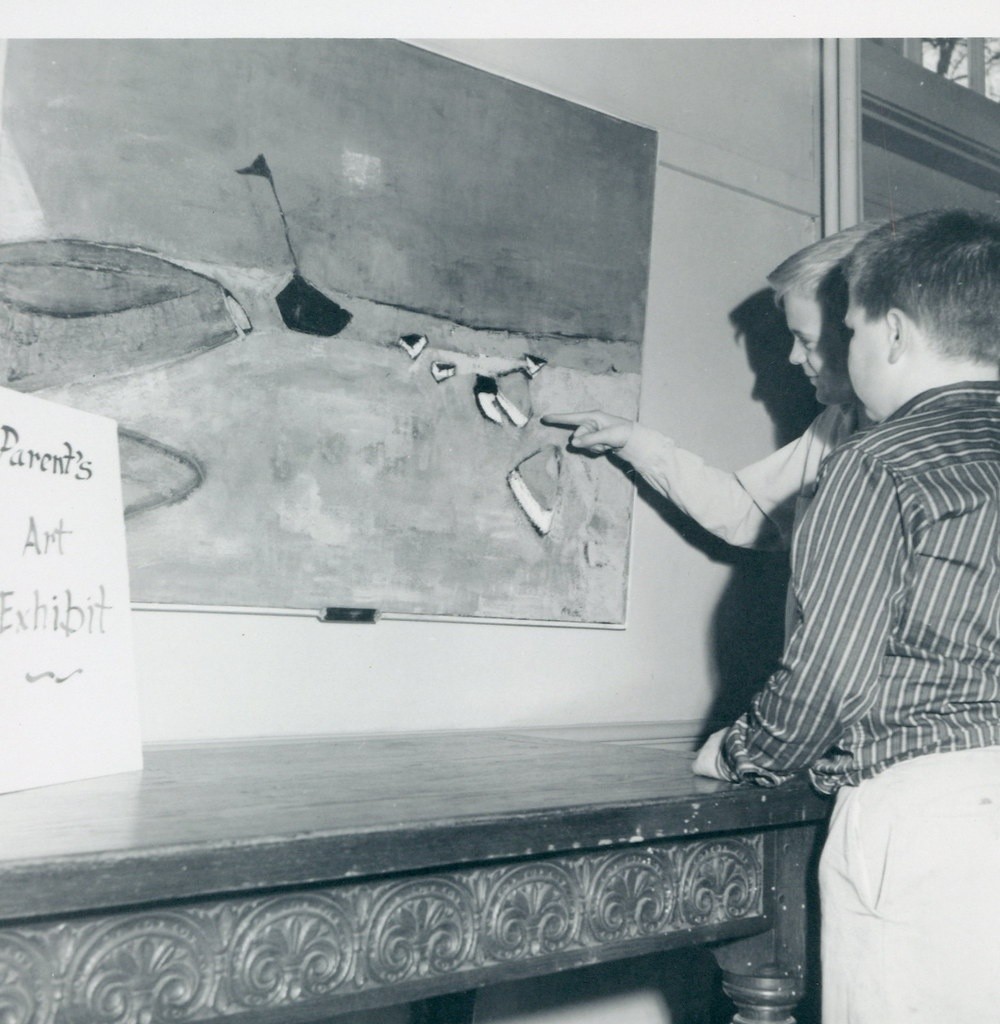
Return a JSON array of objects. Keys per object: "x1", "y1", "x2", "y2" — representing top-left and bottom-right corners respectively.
[{"x1": 0, "y1": 37, "x2": 659, "y2": 632}]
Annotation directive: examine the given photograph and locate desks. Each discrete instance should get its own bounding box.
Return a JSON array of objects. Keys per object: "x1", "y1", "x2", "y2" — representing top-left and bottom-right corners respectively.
[{"x1": 0, "y1": 728, "x2": 832, "y2": 1024}]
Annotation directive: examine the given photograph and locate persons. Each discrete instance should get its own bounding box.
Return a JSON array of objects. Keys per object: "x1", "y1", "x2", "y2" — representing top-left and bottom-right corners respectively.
[
  {"x1": 542, "y1": 215, "x2": 903, "y2": 555},
  {"x1": 692, "y1": 207, "x2": 1000, "y2": 1024}
]
